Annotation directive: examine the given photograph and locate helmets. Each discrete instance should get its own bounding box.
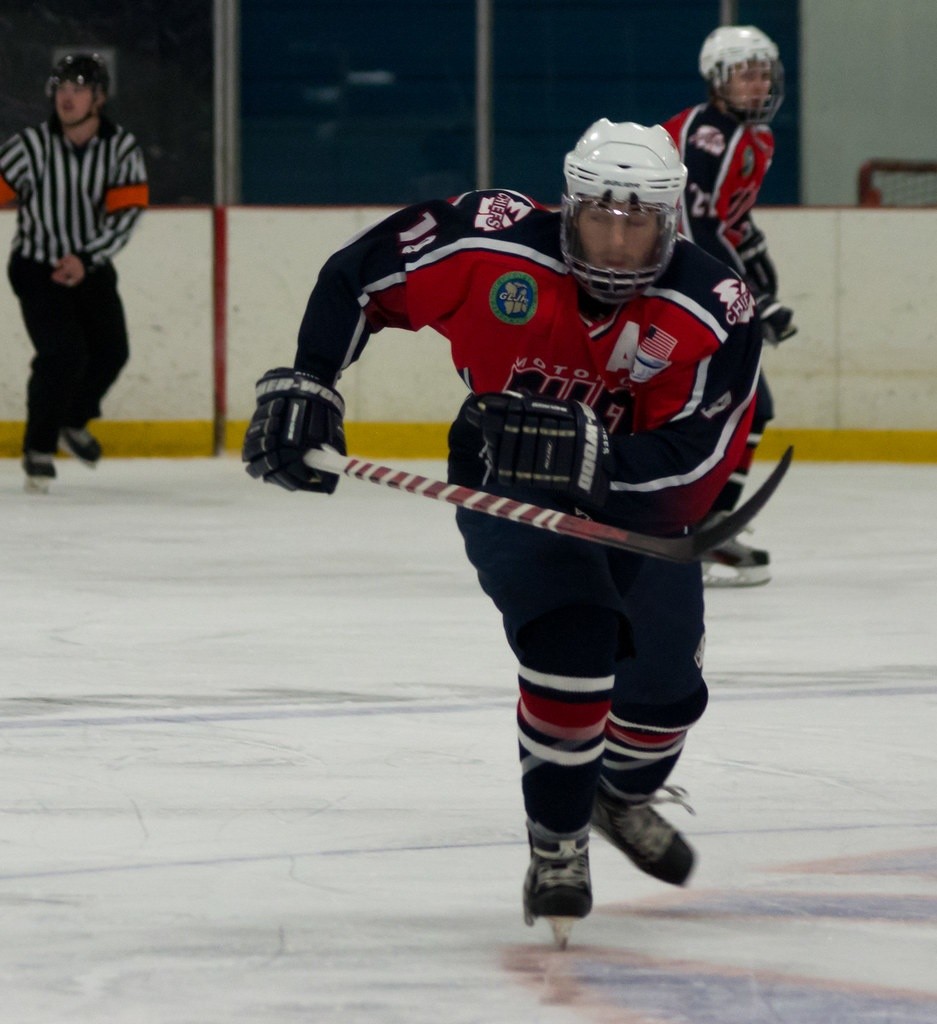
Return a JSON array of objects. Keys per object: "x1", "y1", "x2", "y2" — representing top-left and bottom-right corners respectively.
[
  {"x1": 697, "y1": 25, "x2": 784, "y2": 124},
  {"x1": 563, "y1": 117, "x2": 688, "y2": 304},
  {"x1": 50, "y1": 52, "x2": 109, "y2": 93}
]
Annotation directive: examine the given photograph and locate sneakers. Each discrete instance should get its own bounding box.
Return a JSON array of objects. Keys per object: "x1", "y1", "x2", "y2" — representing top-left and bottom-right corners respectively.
[
  {"x1": 22, "y1": 450, "x2": 57, "y2": 495},
  {"x1": 695, "y1": 511, "x2": 772, "y2": 587},
  {"x1": 589, "y1": 775, "x2": 697, "y2": 886},
  {"x1": 521, "y1": 817, "x2": 593, "y2": 951},
  {"x1": 57, "y1": 425, "x2": 102, "y2": 472}
]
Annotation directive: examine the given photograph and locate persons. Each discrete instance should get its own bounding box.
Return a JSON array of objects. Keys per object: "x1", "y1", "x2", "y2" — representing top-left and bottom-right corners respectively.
[
  {"x1": 655, "y1": 26, "x2": 797, "y2": 586},
  {"x1": 241, "y1": 116, "x2": 764, "y2": 947},
  {"x1": 0, "y1": 52, "x2": 149, "y2": 496}
]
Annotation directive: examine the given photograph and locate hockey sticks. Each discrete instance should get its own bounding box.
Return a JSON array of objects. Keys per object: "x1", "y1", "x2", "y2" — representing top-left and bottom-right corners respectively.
[{"x1": 304, "y1": 445, "x2": 798, "y2": 565}]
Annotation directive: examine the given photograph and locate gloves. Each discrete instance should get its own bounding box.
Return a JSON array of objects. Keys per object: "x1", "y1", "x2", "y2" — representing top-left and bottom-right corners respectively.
[
  {"x1": 241, "y1": 368, "x2": 346, "y2": 494},
  {"x1": 735, "y1": 230, "x2": 777, "y2": 301},
  {"x1": 757, "y1": 294, "x2": 792, "y2": 340},
  {"x1": 464, "y1": 388, "x2": 611, "y2": 514}
]
